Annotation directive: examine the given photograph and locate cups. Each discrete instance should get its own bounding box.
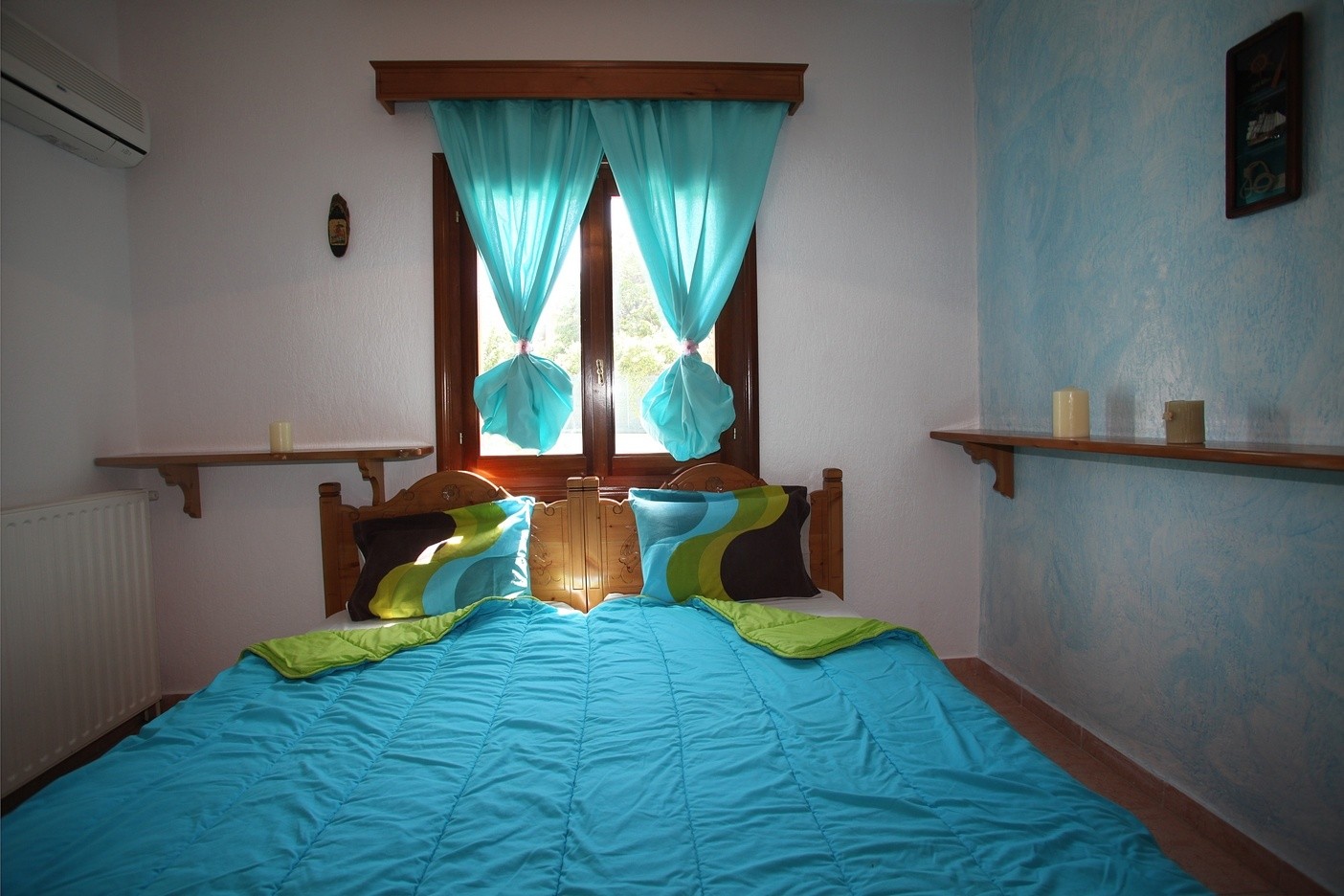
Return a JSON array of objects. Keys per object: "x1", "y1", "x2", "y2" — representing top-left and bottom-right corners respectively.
[{"x1": 1164, "y1": 400, "x2": 1206, "y2": 443}]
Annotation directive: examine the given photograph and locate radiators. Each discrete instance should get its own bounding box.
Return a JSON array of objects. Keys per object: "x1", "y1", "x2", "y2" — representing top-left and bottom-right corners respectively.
[{"x1": 1, "y1": 485, "x2": 168, "y2": 799}]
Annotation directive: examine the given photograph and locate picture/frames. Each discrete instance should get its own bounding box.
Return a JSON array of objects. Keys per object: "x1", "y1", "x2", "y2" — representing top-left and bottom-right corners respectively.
[{"x1": 1226, "y1": 10, "x2": 1303, "y2": 220}]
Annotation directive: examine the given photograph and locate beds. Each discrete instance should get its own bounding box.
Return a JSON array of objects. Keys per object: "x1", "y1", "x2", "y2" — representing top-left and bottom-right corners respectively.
[{"x1": 0, "y1": 460, "x2": 1225, "y2": 896}]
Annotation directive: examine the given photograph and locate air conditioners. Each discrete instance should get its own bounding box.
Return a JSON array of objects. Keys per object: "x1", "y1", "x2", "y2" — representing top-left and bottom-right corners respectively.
[{"x1": 0, "y1": 3, "x2": 153, "y2": 169}]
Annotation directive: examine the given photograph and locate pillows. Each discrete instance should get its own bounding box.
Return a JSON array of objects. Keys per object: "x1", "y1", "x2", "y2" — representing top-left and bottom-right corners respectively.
[
  {"x1": 345, "y1": 495, "x2": 535, "y2": 622},
  {"x1": 628, "y1": 484, "x2": 822, "y2": 604}
]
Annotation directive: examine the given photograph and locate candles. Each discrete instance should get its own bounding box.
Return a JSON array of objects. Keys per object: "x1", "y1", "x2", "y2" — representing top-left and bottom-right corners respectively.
[
  {"x1": 1053, "y1": 386, "x2": 1090, "y2": 439},
  {"x1": 1161, "y1": 399, "x2": 1206, "y2": 444},
  {"x1": 268, "y1": 420, "x2": 294, "y2": 453}
]
